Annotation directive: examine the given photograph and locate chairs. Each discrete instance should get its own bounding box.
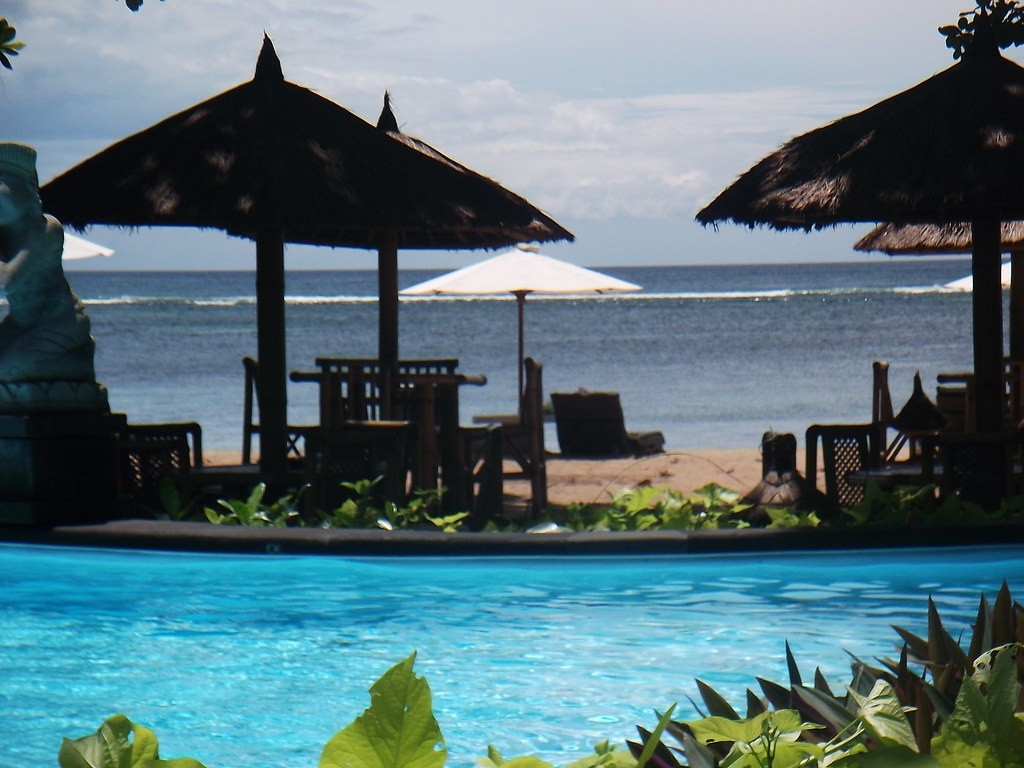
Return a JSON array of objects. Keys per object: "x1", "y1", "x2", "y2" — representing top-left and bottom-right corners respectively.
[
  {"x1": 110, "y1": 422, "x2": 202, "y2": 503},
  {"x1": 312, "y1": 356, "x2": 418, "y2": 506},
  {"x1": 397, "y1": 359, "x2": 460, "y2": 375},
  {"x1": 462, "y1": 356, "x2": 546, "y2": 518},
  {"x1": 242, "y1": 357, "x2": 317, "y2": 464},
  {"x1": 806, "y1": 424, "x2": 880, "y2": 504}
]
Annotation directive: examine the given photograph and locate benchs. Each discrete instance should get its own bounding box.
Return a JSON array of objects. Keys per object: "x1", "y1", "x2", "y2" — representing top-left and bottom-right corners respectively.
[{"x1": 549, "y1": 390, "x2": 665, "y2": 462}]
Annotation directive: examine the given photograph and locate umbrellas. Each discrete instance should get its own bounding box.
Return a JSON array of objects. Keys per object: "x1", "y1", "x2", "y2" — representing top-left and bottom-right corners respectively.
[
  {"x1": 40, "y1": 35, "x2": 575, "y2": 477},
  {"x1": 398, "y1": 243, "x2": 643, "y2": 407},
  {"x1": 695, "y1": 50, "x2": 1024, "y2": 511}
]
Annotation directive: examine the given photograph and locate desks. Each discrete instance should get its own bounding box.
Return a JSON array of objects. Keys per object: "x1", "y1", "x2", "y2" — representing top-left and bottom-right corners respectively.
[
  {"x1": 472, "y1": 414, "x2": 516, "y2": 425},
  {"x1": 290, "y1": 370, "x2": 488, "y2": 503},
  {"x1": 848, "y1": 467, "x2": 941, "y2": 489}
]
